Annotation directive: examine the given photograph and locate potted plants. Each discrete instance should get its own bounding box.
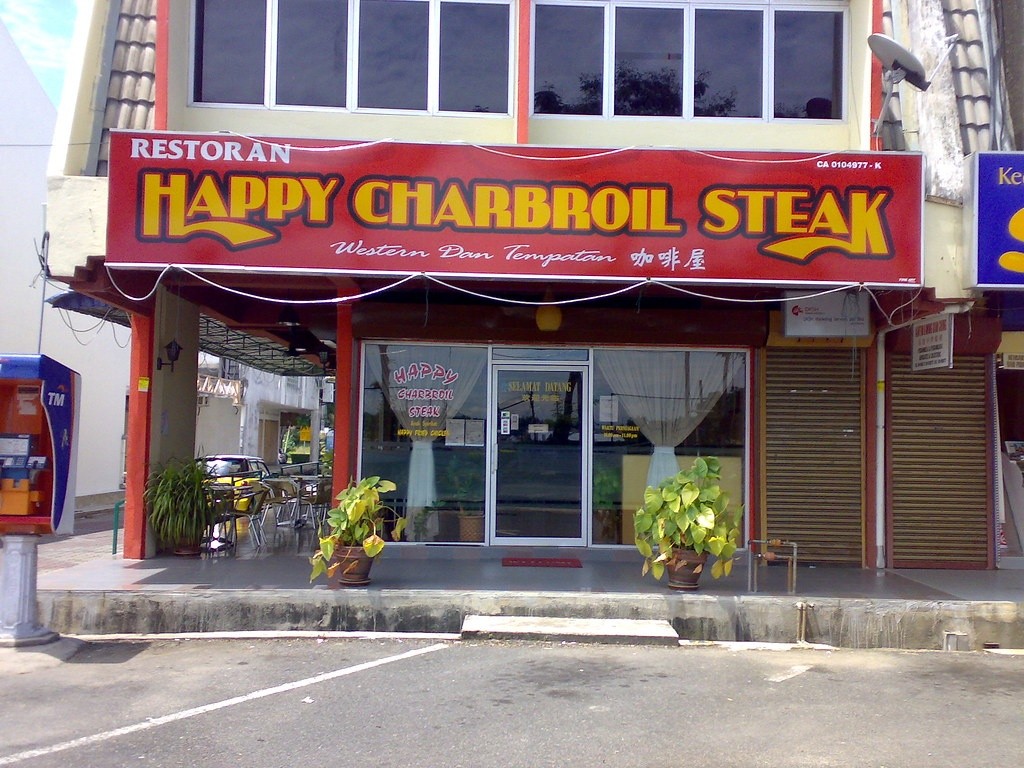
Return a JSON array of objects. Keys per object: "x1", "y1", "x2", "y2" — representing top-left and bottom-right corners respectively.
[
  {"x1": 142, "y1": 443, "x2": 227, "y2": 556},
  {"x1": 414, "y1": 456, "x2": 487, "y2": 542},
  {"x1": 310, "y1": 476, "x2": 407, "y2": 588},
  {"x1": 632, "y1": 455, "x2": 746, "y2": 592}
]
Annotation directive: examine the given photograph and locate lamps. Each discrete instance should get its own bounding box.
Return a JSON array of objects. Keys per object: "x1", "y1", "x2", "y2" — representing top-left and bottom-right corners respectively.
[
  {"x1": 318, "y1": 341, "x2": 336, "y2": 379},
  {"x1": 534, "y1": 289, "x2": 563, "y2": 331},
  {"x1": 157, "y1": 339, "x2": 183, "y2": 373}
]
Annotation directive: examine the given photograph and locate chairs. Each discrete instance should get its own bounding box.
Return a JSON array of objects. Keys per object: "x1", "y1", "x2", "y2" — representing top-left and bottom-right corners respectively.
[{"x1": 208, "y1": 475, "x2": 333, "y2": 554}]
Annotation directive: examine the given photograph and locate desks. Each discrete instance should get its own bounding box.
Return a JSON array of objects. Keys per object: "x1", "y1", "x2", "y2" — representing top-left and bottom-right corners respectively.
[
  {"x1": 262, "y1": 478, "x2": 328, "y2": 530},
  {"x1": 198, "y1": 485, "x2": 254, "y2": 551}
]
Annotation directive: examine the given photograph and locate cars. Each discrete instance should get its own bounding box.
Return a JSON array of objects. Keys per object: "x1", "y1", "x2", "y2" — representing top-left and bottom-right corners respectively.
[{"x1": 195, "y1": 454, "x2": 274, "y2": 515}]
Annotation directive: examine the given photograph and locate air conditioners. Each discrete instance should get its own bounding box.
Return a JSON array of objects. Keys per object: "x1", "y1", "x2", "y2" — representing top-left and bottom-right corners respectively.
[{"x1": 322, "y1": 380, "x2": 335, "y2": 403}]
